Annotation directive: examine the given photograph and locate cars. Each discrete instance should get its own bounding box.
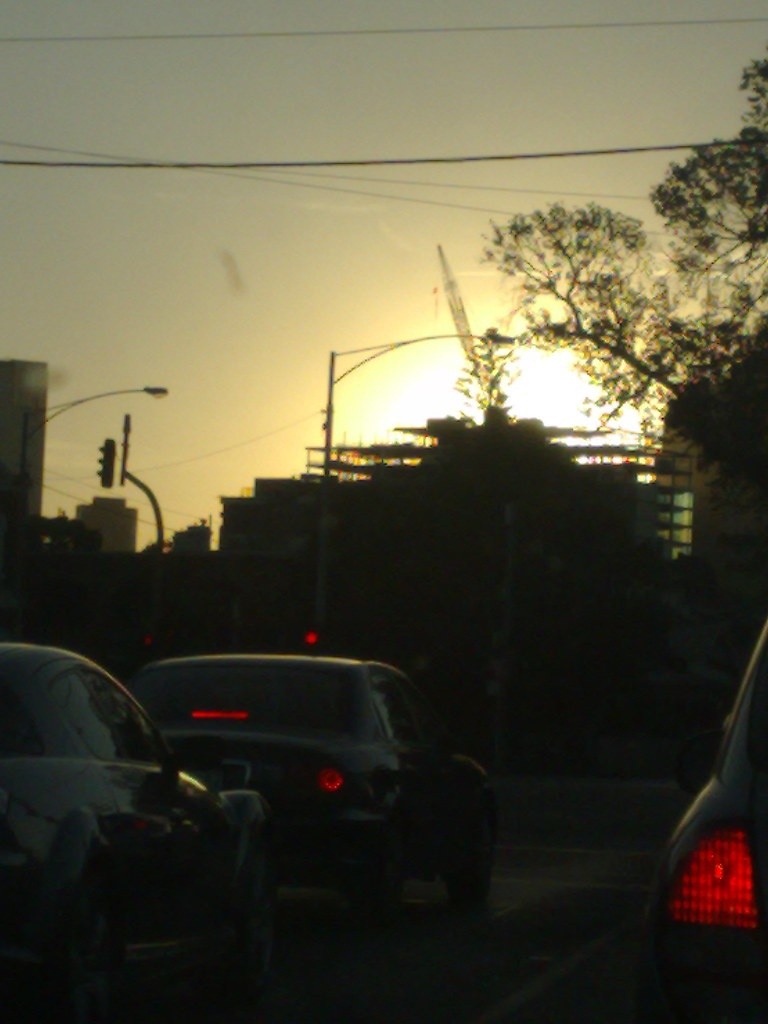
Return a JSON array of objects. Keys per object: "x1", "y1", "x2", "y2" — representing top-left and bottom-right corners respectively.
[
  {"x1": 630, "y1": 616, "x2": 767, "y2": 1023},
  {"x1": 0, "y1": 638, "x2": 282, "y2": 1024},
  {"x1": 123, "y1": 654, "x2": 498, "y2": 924}
]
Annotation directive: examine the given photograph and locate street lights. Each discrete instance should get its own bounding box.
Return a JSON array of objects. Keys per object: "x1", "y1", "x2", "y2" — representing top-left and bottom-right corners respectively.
[{"x1": 313, "y1": 332, "x2": 515, "y2": 652}]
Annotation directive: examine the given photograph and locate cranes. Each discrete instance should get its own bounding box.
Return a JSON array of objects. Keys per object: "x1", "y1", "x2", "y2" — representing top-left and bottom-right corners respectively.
[{"x1": 429, "y1": 237, "x2": 479, "y2": 361}]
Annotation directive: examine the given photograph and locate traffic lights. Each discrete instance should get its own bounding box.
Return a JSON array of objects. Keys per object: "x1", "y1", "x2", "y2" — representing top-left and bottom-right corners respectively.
[
  {"x1": 13, "y1": 383, "x2": 170, "y2": 644},
  {"x1": 301, "y1": 625, "x2": 325, "y2": 650},
  {"x1": 96, "y1": 438, "x2": 115, "y2": 490}
]
